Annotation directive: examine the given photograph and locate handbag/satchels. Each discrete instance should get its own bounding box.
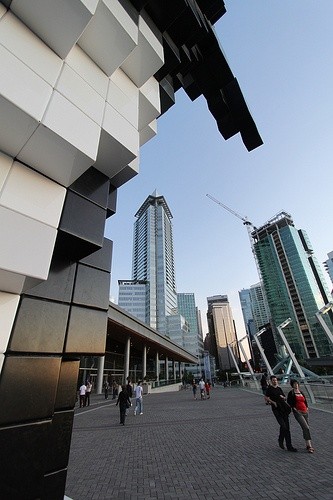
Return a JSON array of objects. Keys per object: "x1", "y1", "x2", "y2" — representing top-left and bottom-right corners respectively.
[{"x1": 281, "y1": 401, "x2": 291, "y2": 415}]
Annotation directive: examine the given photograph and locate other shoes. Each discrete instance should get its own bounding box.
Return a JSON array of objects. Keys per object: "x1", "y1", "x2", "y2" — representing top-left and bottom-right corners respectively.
[
  {"x1": 287, "y1": 447, "x2": 297, "y2": 452},
  {"x1": 193, "y1": 396, "x2": 211, "y2": 401},
  {"x1": 278, "y1": 440, "x2": 285, "y2": 449},
  {"x1": 119, "y1": 422, "x2": 125, "y2": 426},
  {"x1": 139, "y1": 413, "x2": 144, "y2": 415},
  {"x1": 134, "y1": 411, "x2": 136, "y2": 416}
]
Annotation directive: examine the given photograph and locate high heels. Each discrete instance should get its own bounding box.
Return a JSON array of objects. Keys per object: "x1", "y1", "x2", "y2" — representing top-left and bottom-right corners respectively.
[{"x1": 265, "y1": 402, "x2": 271, "y2": 406}]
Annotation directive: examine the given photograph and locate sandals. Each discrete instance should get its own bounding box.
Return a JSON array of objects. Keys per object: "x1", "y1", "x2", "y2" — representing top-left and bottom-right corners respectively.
[{"x1": 306, "y1": 445, "x2": 314, "y2": 453}]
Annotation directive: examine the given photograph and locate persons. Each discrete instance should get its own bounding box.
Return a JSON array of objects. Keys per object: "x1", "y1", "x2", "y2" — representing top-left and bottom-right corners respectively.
[
  {"x1": 191, "y1": 377, "x2": 216, "y2": 400},
  {"x1": 223, "y1": 380, "x2": 227, "y2": 391},
  {"x1": 104, "y1": 374, "x2": 143, "y2": 425},
  {"x1": 79, "y1": 379, "x2": 93, "y2": 408},
  {"x1": 259, "y1": 373, "x2": 316, "y2": 454}
]
